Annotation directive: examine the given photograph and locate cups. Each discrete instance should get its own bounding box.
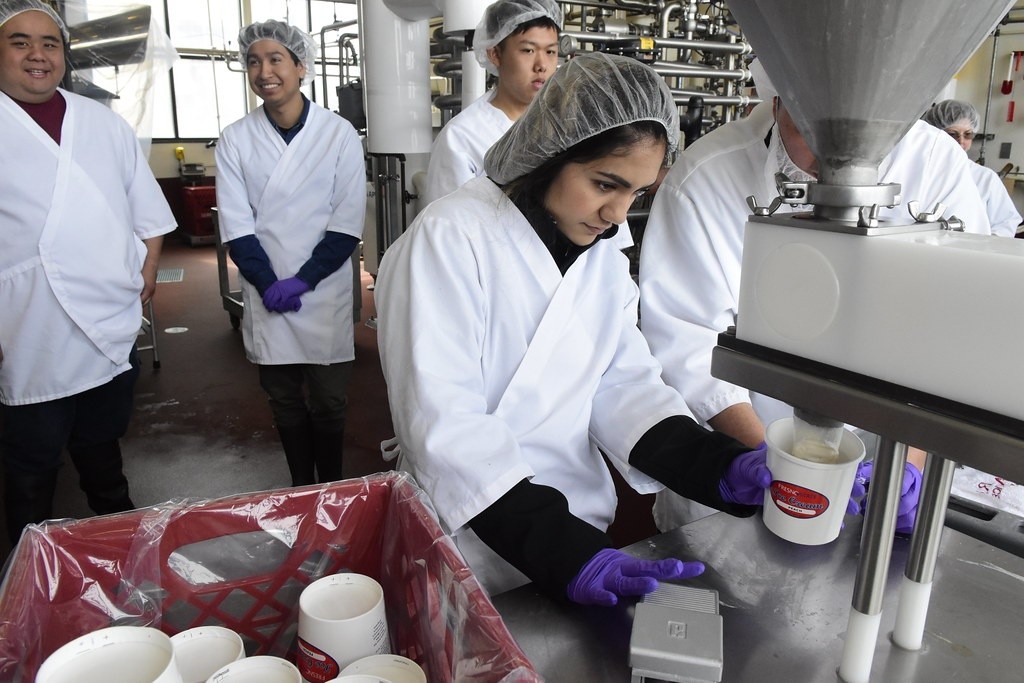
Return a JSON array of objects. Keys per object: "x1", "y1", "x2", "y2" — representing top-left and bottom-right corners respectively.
[
  {"x1": 29, "y1": 573, "x2": 427, "y2": 683},
  {"x1": 762, "y1": 416, "x2": 866, "y2": 545}
]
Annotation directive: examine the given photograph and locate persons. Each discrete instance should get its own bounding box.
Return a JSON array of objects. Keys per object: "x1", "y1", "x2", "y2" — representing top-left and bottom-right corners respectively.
[
  {"x1": 0, "y1": 0, "x2": 180, "y2": 566},
  {"x1": 375, "y1": 52, "x2": 867, "y2": 610},
  {"x1": 214, "y1": 19, "x2": 367, "y2": 488},
  {"x1": 420, "y1": 0, "x2": 564, "y2": 205},
  {"x1": 637, "y1": 57, "x2": 1023, "y2": 539}
]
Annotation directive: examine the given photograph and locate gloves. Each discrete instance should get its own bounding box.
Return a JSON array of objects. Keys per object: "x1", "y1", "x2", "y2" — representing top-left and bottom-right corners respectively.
[
  {"x1": 719, "y1": 441, "x2": 865, "y2": 531},
  {"x1": 263, "y1": 276, "x2": 310, "y2": 313},
  {"x1": 858, "y1": 459, "x2": 922, "y2": 534},
  {"x1": 567, "y1": 548, "x2": 705, "y2": 607}
]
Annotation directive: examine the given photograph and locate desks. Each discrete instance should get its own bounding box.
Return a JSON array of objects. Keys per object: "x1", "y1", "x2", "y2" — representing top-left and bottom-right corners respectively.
[{"x1": 483, "y1": 505, "x2": 1024, "y2": 683}]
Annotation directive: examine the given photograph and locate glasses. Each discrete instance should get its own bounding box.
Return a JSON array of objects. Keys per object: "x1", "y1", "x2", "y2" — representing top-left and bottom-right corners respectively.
[{"x1": 949, "y1": 132, "x2": 977, "y2": 141}]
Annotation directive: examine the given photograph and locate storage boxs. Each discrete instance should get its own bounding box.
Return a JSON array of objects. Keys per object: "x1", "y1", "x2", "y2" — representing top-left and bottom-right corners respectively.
[{"x1": 0, "y1": 467, "x2": 548, "y2": 683}]
